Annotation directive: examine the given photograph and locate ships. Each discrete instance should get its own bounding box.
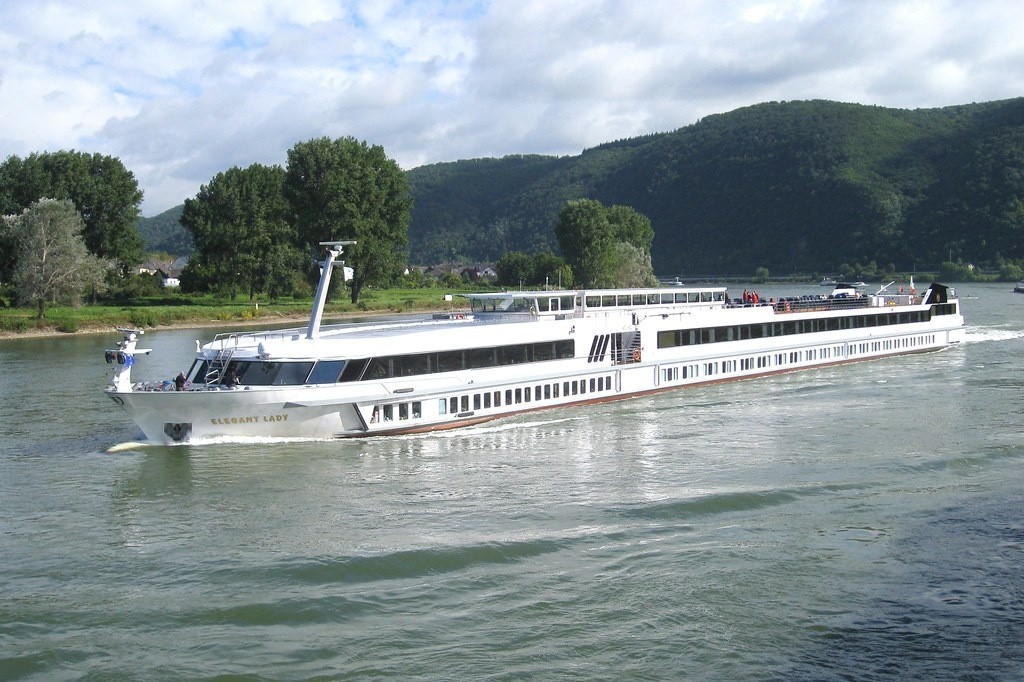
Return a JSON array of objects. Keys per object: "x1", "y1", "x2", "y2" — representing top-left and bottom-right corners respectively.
[{"x1": 102, "y1": 239, "x2": 964, "y2": 450}]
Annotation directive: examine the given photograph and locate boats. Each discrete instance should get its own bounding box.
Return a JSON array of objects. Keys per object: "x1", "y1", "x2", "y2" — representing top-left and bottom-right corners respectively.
[
  {"x1": 821, "y1": 277, "x2": 837, "y2": 286},
  {"x1": 1014, "y1": 282, "x2": 1024, "y2": 293},
  {"x1": 669, "y1": 277, "x2": 683, "y2": 285},
  {"x1": 850, "y1": 281, "x2": 871, "y2": 288}
]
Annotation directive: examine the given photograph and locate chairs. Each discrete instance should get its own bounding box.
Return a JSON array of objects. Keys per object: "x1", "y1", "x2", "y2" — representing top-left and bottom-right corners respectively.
[{"x1": 730, "y1": 293, "x2": 868, "y2": 311}]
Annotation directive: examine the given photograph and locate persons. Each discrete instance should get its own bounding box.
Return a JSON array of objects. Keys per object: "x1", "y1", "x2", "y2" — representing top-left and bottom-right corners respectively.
[
  {"x1": 175, "y1": 371, "x2": 192, "y2": 391},
  {"x1": 226, "y1": 368, "x2": 238, "y2": 390},
  {"x1": 695, "y1": 292, "x2": 728, "y2": 309},
  {"x1": 822, "y1": 291, "x2": 859, "y2": 300},
  {"x1": 743, "y1": 288, "x2": 759, "y2": 308}
]
